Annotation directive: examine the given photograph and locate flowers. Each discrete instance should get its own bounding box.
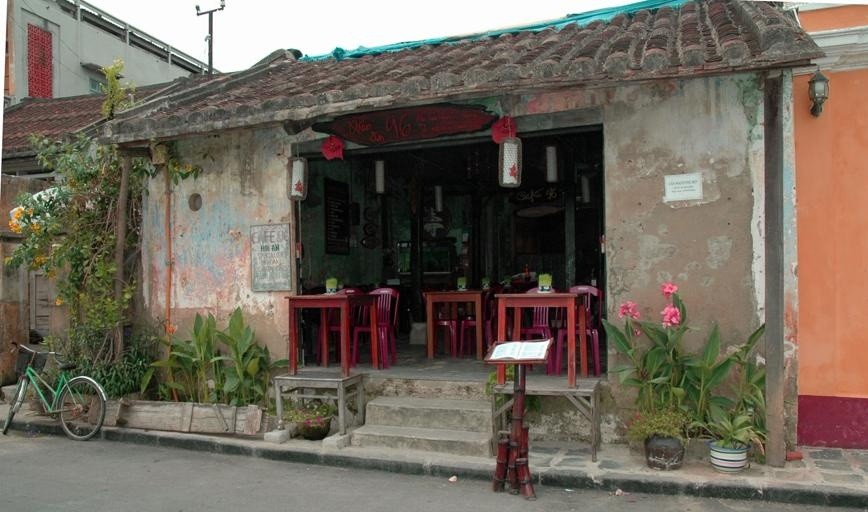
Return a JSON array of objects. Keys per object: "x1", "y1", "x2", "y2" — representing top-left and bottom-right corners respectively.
[
  {"x1": 599, "y1": 281, "x2": 768, "y2": 435},
  {"x1": 285, "y1": 401, "x2": 335, "y2": 425},
  {"x1": 621, "y1": 406, "x2": 693, "y2": 445}
]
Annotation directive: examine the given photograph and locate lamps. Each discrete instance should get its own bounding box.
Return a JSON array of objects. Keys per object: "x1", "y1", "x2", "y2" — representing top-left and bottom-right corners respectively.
[{"x1": 807, "y1": 64, "x2": 831, "y2": 116}]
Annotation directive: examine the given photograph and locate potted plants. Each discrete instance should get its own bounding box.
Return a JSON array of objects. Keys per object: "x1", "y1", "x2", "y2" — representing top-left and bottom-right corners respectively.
[
  {"x1": 707, "y1": 411, "x2": 770, "y2": 474},
  {"x1": 538, "y1": 273, "x2": 553, "y2": 292}
]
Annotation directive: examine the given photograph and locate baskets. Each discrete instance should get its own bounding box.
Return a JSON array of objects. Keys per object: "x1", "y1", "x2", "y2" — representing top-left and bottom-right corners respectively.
[{"x1": 14, "y1": 350, "x2": 47, "y2": 376}]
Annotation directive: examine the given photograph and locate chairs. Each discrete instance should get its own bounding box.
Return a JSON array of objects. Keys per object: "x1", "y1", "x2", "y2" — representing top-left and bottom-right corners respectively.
[
  {"x1": 315, "y1": 287, "x2": 400, "y2": 369},
  {"x1": 516, "y1": 284, "x2": 604, "y2": 378},
  {"x1": 431, "y1": 286, "x2": 504, "y2": 359}
]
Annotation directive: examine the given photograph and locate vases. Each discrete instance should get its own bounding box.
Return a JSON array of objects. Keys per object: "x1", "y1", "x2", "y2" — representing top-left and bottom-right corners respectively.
[
  {"x1": 295, "y1": 416, "x2": 332, "y2": 440},
  {"x1": 643, "y1": 434, "x2": 685, "y2": 469}
]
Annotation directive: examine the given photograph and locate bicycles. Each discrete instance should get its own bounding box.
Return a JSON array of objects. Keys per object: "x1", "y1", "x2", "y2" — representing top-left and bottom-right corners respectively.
[{"x1": 2, "y1": 340, "x2": 107, "y2": 442}]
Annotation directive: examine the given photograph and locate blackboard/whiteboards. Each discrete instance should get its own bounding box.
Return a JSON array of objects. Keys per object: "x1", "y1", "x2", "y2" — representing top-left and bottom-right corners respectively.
[{"x1": 248, "y1": 222, "x2": 292, "y2": 292}]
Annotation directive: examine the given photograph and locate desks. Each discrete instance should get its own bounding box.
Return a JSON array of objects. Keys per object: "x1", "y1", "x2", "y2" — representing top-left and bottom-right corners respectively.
[
  {"x1": 421, "y1": 288, "x2": 487, "y2": 361},
  {"x1": 493, "y1": 291, "x2": 589, "y2": 389},
  {"x1": 272, "y1": 369, "x2": 366, "y2": 436},
  {"x1": 285, "y1": 292, "x2": 382, "y2": 377},
  {"x1": 489, "y1": 376, "x2": 603, "y2": 462}
]
